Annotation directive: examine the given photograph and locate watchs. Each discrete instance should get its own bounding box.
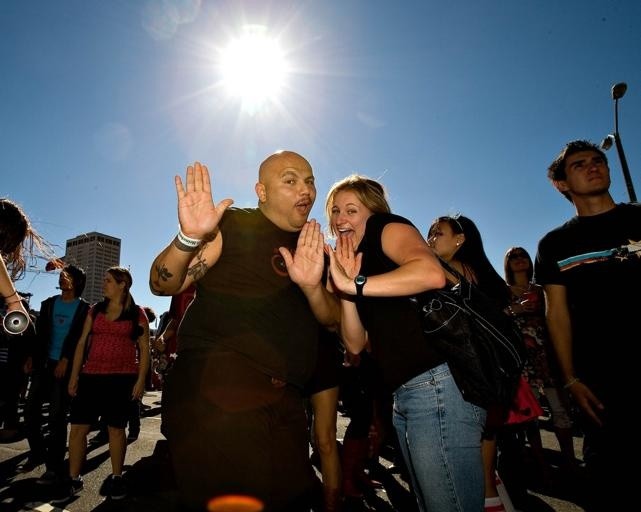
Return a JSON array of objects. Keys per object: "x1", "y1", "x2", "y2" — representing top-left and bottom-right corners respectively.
[{"x1": 354, "y1": 274, "x2": 367, "y2": 296}]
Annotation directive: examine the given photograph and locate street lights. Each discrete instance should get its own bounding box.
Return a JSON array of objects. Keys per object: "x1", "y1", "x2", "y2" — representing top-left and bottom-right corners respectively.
[{"x1": 597, "y1": 79, "x2": 639, "y2": 203}]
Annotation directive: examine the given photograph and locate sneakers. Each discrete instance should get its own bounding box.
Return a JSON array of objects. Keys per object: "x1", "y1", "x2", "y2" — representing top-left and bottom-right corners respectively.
[
  {"x1": 128, "y1": 431, "x2": 138, "y2": 440},
  {"x1": 89, "y1": 433, "x2": 109, "y2": 444},
  {"x1": 111, "y1": 475, "x2": 124, "y2": 499},
  {"x1": 36, "y1": 467, "x2": 83, "y2": 503}
]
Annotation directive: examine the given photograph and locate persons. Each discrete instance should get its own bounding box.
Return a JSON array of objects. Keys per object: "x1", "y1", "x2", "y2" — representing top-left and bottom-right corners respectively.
[
  {"x1": 1, "y1": 292, "x2": 44, "y2": 439},
  {"x1": 38, "y1": 265, "x2": 176, "y2": 498},
  {"x1": 498, "y1": 243, "x2": 584, "y2": 484},
  {"x1": 1, "y1": 197, "x2": 38, "y2": 345},
  {"x1": 148, "y1": 150, "x2": 344, "y2": 511},
  {"x1": 531, "y1": 140, "x2": 641, "y2": 511},
  {"x1": 323, "y1": 174, "x2": 494, "y2": 510},
  {"x1": 415, "y1": 215, "x2": 510, "y2": 499}
]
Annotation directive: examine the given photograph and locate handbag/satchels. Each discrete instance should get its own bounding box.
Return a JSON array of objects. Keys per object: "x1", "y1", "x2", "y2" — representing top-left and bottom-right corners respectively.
[{"x1": 409, "y1": 251, "x2": 527, "y2": 409}]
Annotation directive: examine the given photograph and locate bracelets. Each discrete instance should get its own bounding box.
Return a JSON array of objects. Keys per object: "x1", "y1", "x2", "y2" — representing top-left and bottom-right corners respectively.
[
  {"x1": 560, "y1": 376, "x2": 579, "y2": 389},
  {"x1": 177, "y1": 229, "x2": 204, "y2": 248}
]
